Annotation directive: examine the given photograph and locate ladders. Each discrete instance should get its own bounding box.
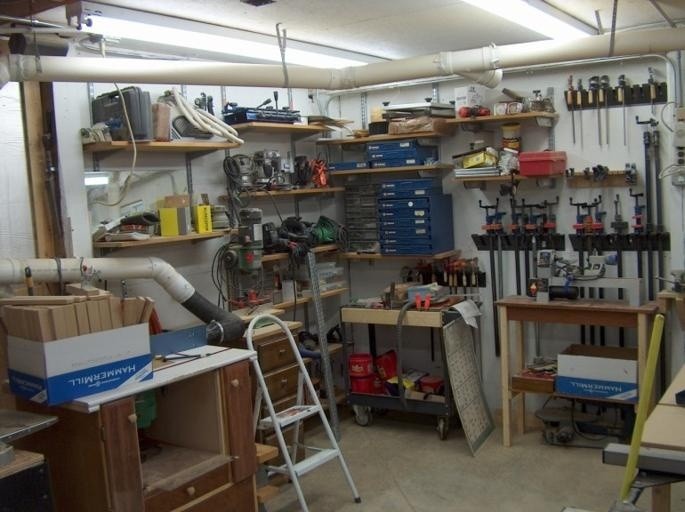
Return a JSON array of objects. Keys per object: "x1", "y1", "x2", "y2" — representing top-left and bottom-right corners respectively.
[{"x1": 246, "y1": 313, "x2": 361, "y2": 512}]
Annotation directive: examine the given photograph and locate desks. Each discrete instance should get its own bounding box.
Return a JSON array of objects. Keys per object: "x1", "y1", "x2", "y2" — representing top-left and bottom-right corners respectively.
[{"x1": 496, "y1": 293, "x2": 659, "y2": 448}]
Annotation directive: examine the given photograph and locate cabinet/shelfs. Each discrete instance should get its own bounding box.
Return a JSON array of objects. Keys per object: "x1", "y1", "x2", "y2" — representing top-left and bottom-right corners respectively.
[
  {"x1": 235, "y1": 320, "x2": 305, "y2": 487},
  {"x1": 13, "y1": 341, "x2": 256, "y2": 512}
]
